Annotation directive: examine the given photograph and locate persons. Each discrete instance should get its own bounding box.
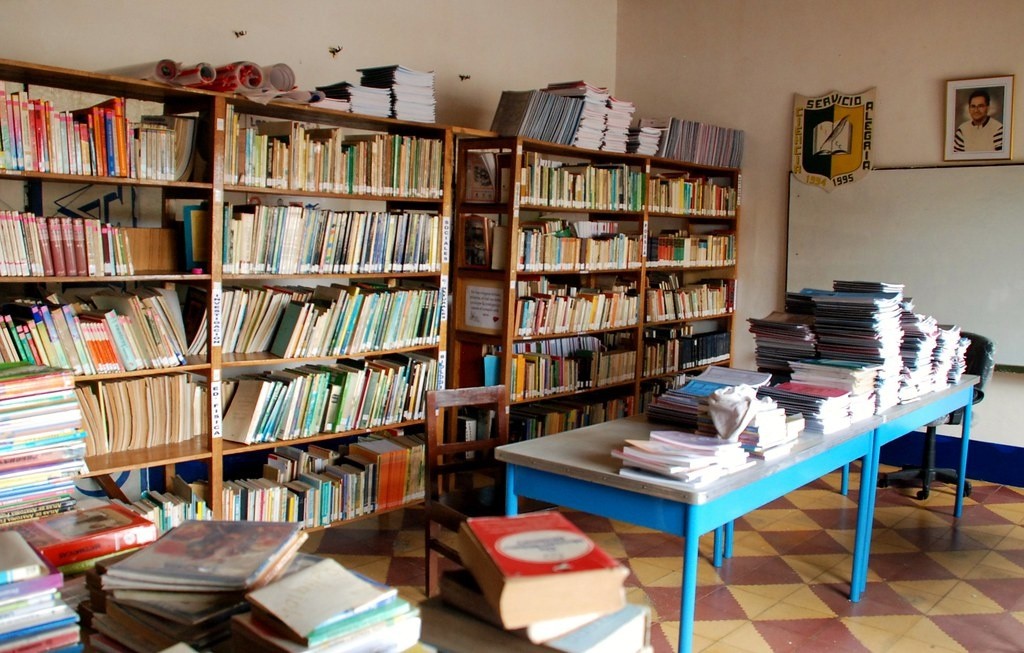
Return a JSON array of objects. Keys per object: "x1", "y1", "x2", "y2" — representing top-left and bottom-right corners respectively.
[{"x1": 955, "y1": 89, "x2": 1002, "y2": 153}]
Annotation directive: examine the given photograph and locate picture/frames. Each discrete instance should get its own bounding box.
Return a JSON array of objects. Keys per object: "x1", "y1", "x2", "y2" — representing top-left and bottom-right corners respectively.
[{"x1": 941, "y1": 74, "x2": 1016, "y2": 162}]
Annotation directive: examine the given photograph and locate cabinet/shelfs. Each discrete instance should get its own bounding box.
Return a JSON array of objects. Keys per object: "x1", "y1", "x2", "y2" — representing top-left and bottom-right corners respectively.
[{"x1": 0, "y1": 53, "x2": 744, "y2": 535}]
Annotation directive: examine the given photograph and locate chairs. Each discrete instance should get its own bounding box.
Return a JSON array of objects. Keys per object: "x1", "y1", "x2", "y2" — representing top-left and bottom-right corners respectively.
[
  {"x1": 877, "y1": 331, "x2": 997, "y2": 500},
  {"x1": 423, "y1": 382, "x2": 560, "y2": 598}
]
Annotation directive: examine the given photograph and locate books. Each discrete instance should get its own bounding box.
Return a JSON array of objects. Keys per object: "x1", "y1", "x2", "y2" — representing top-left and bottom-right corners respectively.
[
  {"x1": 813, "y1": 120, "x2": 852, "y2": 155},
  {"x1": 0, "y1": 63, "x2": 971, "y2": 653}
]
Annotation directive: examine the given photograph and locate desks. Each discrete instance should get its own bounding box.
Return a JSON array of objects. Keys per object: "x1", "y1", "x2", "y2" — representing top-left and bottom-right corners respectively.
[{"x1": 492, "y1": 374, "x2": 981, "y2": 653}]
